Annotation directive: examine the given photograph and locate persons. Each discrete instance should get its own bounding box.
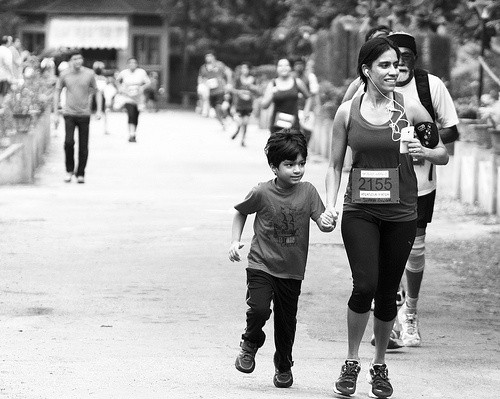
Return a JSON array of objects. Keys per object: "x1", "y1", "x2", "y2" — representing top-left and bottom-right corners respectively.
[
  {"x1": 197, "y1": 51, "x2": 320, "y2": 147},
  {"x1": 339, "y1": 24, "x2": 460, "y2": 349},
  {"x1": 53, "y1": 49, "x2": 103, "y2": 184},
  {"x1": 320, "y1": 36, "x2": 449, "y2": 399},
  {"x1": 227, "y1": 127, "x2": 336, "y2": 389},
  {"x1": 0, "y1": 35, "x2": 159, "y2": 143}
]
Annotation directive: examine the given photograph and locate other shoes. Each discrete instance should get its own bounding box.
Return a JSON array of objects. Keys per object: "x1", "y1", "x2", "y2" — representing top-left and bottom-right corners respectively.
[
  {"x1": 64, "y1": 171, "x2": 73, "y2": 182},
  {"x1": 77, "y1": 174, "x2": 83, "y2": 182}
]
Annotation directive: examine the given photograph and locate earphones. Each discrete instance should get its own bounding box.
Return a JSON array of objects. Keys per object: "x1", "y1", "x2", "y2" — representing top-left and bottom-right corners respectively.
[{"x1": 365, "y1": 69, "x2": 369, "y2": 76}]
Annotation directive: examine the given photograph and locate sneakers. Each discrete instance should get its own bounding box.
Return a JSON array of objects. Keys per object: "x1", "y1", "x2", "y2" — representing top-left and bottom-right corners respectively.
[
  {"x1": 396, "y1": 282, "x2": 405, "y2": 305},
  {"x1": 273, "y1": 357, "x2": 293, "y2": 388},
  {"x1": 235, "y1": 339, "x2": 258, "y2": 373},
  {"x1": 370, "y1": 328, "x2": 400, "y2": 349},
  {"x1": 371, "y1": 298, "x2": 376, "y2": 311},
  {"x1": 397, "y1": 303, "x2": 422, "y2": 346},
  {"x1": 368, "y1": 361, "x2": 393, "y2": 397},
  {"x1": 333, "y1": 359, "x2": 361, "y2": 396}
]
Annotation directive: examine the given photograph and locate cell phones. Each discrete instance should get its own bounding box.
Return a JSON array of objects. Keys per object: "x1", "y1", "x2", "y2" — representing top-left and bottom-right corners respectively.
[{"x1": 400, "y1": 126, "x2": 414, "y2": 154}]
